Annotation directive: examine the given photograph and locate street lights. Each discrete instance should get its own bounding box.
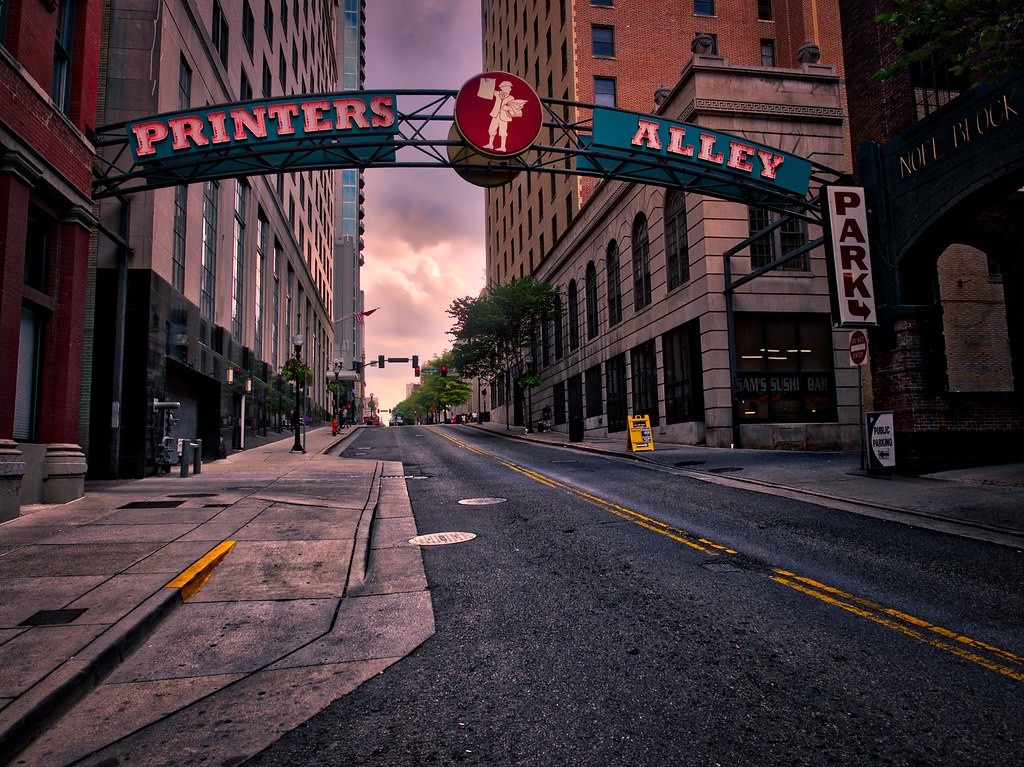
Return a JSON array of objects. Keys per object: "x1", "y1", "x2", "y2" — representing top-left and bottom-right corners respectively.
[
  {"x1": 525, "y1": 354, "x2": 535, "y2": 433},
  {"x1": 333, "y1": 358, "x2": 344, "y2": 431},
  {"x1": 370, "y1": 393, "x2": 374, "y2": 420},
  {"x1": 291, "y1": 333, "x2": 304, "y2": 451}
]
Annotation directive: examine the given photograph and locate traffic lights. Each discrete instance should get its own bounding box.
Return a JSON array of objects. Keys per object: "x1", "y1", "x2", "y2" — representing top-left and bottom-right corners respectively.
[
  {"x1": 348, "y1": 403, "x2": 351, "y2": 408},
  {"x1": 415, "y1": 366, "x2": 420, "y2": 377},
  {"x1": 441, "y1": 365, "x2": 446, "y2": 375}
]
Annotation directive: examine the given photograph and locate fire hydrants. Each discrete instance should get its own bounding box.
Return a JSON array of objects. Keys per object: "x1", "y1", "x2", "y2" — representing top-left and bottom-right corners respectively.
[{"x1": 332, "y1": 419, "x2": 338, "y2": 434}]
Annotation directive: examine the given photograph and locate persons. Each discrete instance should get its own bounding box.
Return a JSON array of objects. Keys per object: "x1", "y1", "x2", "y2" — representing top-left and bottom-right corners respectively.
[
  {"x1": 393, "y1": 415, "x2": 398, "y2": 426},
  {"x1": 339, "y1": 405, "x2": 347, "y2": 429},
  {"x1": 462, "y1": 411, "x2": 478, "y2": 425},
  {"x1": 541, "y1": 403, "x2": 551, "y2": 432}
]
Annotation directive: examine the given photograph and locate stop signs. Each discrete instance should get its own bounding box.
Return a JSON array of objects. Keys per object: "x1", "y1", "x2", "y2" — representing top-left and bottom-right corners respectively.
[{"x1": 848, "y1": 329, "x2": 869, "y2": 366}]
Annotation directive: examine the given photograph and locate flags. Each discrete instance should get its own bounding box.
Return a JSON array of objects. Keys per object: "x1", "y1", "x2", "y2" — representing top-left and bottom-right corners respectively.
[{"x1": 355, "y1": 311, "x2": 365, "y2": 325}]
